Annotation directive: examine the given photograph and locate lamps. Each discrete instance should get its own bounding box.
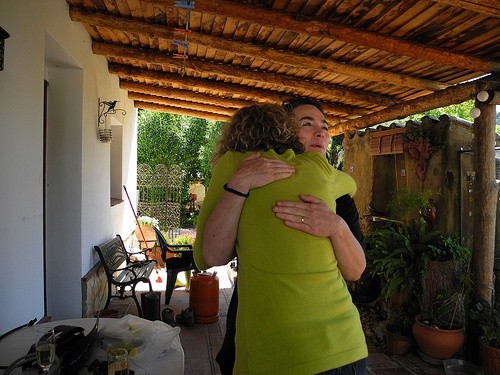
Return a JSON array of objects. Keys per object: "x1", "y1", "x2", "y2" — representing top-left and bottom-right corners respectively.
[{"x1": 98, "y1": 97, "x2": 125, "y2": 143}]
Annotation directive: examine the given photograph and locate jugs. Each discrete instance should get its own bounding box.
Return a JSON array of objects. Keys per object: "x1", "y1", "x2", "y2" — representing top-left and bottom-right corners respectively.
[{"x1": 180, "y1": 307, "x2": 194, "y2": 327}]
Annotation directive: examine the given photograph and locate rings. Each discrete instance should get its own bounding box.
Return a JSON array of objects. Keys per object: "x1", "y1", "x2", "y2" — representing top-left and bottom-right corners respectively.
[{"x1": 301, "y1": 216, "x2": 304, "y2": 223}]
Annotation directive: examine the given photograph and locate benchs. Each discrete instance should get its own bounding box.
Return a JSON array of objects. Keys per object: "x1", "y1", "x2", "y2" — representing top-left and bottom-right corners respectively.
[{"x1": 94, "y1": 235, "x2": 157, "y2": 317}]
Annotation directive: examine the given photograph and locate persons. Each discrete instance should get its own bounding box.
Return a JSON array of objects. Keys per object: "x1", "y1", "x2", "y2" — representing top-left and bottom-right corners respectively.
[{"x1": 192, "y1": 98, "x2": 369, "y2": 375}]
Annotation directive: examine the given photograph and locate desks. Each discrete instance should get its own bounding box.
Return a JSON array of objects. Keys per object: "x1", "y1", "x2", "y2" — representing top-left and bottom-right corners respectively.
[{"x1": 0, "y1": 318, "x2": 184, "y2": 375}]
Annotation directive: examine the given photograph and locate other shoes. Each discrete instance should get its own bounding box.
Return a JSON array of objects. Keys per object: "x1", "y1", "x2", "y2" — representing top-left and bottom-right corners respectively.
[
  {"x1": 176, "y1": 308, "x2": 194, "y2": 326},
  {"x1": 161, "y1": 308, "x2": 175, "y2": 326}
]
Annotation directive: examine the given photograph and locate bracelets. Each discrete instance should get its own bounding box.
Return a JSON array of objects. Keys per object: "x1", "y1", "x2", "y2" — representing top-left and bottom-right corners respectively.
[{"x1": 224, "y1": 183, "x2": 251, "y2": 197}]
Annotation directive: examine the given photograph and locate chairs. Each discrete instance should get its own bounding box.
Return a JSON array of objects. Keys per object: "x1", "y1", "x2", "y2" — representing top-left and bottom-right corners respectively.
[{"x1": 152, "y1": 226, "x2": 200, "y2": 305}]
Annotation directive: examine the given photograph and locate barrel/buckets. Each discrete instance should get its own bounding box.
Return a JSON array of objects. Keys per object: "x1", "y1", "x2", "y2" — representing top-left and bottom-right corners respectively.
[{"x1": 191, "y1": 271, "x2": 219, "y2": 323}]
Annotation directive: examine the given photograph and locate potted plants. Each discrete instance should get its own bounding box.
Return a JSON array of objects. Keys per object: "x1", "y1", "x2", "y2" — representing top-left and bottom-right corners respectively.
[
  {"x1": 135, "y1": 216, "x2": 158, "y2": 250},
  {"x1": 365, "y1": 225, "x2": 471, "y2": 360},
  {"x1": 470, "y1": 295, "x2": 500, "y2": 375},
  {"x1": 389, "y1": 189, "x2": 437, "y2": 224}
]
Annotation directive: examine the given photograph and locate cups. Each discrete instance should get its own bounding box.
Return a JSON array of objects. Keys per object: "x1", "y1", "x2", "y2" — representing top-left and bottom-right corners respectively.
[
  {"x1": 35, "y1": 327, "x2": 55, "y2": 375},
  {"x1": 107, "y1": 348, "x2": 129, "y2": 375}
]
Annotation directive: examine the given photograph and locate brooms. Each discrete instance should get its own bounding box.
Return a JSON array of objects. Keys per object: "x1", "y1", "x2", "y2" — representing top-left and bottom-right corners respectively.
[{"x1": 122, "y1": 185, "x2": 163, "y2": 284}]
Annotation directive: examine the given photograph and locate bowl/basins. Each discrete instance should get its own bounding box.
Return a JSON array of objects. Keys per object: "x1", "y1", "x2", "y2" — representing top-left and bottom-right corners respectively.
[{"x1": 443, "y1": 359, "x2": 488, "y2": 375}]
